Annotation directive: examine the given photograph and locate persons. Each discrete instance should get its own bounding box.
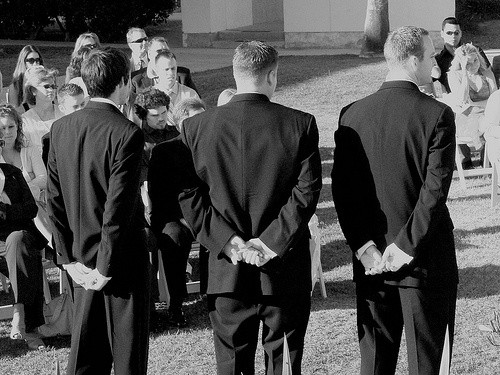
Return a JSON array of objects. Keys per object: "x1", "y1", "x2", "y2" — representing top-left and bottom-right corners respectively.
[
  {"x1": 169, "y1": 41, "x2": 321, "y2": 375},
  {"x1": 431, "y1": 18, "x2": 500, "y2": 194},
  {"x1": 0, "y1": 26, "x2": 235, "y2": 375},
  {"x1": 330, "y1": 24, "x2": 459, "y2": 375}
]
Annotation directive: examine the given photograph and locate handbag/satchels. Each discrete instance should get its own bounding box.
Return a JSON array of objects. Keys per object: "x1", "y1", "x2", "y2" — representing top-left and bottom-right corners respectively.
[{"x1": 38, "y1": 290, "x2": 75, "y2": 337}]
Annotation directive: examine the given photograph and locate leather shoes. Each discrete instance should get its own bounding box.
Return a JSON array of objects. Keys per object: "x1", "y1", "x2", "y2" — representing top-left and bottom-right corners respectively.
[{"x1": 168, "y1": 306, "x2": 188, "y2": 328}]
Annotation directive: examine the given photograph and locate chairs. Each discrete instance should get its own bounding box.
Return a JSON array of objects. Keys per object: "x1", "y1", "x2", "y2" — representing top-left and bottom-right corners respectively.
[
  {"x1": 0, "y1": 241, "x2": 210, "y2": 321},
  {"x1": 433, "y1": 78, "x2": 500, "y2": 209},
  {"x1": 307, "y1": 213, "x2": 329, "y2": 300}
]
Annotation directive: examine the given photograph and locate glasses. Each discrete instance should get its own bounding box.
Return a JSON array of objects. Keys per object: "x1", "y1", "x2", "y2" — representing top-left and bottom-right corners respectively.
[
  {"x1": 0, "y1": 140, "x2": 5, "y2": 148},
  {"x1": 444, "y1": 31, "x2": 461, "y2": 35},
  {"x1": 131, "y1": 37, "x2": 148, "y2": 43},
  {"x1": 37, "y1": 83, "x2": 58, "y2": 89},
  {"x1": 25, "y1": 58, "x2": 41, "y2": 64},
  {"x1": 86, "y1": 44, "x2": 98, "y2": 48}
]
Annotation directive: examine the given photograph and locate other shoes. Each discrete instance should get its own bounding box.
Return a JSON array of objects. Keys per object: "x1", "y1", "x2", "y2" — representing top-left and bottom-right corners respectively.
[
  {"x1": 25, "y1": 330, "x2": 46, "y2": 350},
  {"x1": 11, "y1": 314, "x2": 26, "y2": 339},
  {"x1": 461, "y1": 160, "x2": 472, "y2": 170}
]
